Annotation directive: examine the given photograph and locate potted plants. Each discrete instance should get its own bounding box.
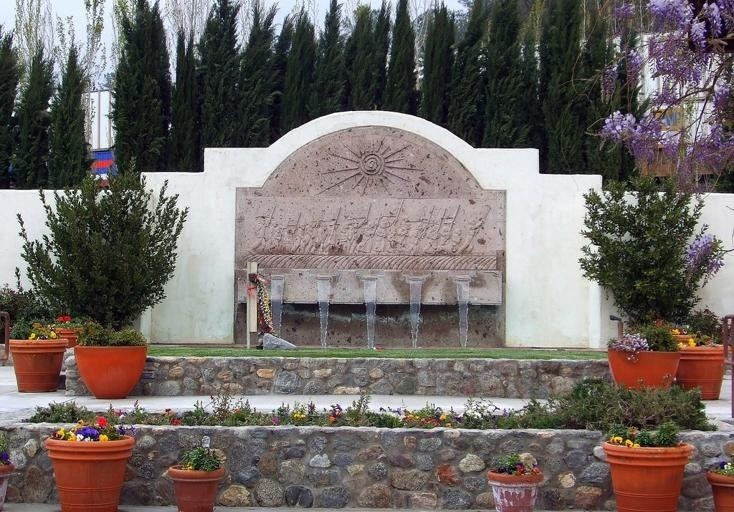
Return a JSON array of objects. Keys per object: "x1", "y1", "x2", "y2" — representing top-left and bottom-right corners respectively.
[
  {"x1": 488, "y1": 460, "x2": 544, "y2": 512},
  {"x1": 54, "y1": 314, "x2": 77, "y2": 348},
  {"x1": 602, "y1": 428, "x2": 694, "y2": 512},
  {"x1": 707, "y1": 461, "x2": 734, "y2": 512},
  {"x1": 675, "y1": 332, "x2": 731, "y2": 401},
  {"x1": 74, "y1": 331, "x2": 148, "y2": 399},
  {"x1": 8, "y1": 325, "x2": 67, "y2": 393},
  {"x1": 0, "y1": 450, "x2": 14, "y2": 512},
  {"x1": 45, "y1": 415, "x2": 135, "y2": 512},
  {"x1": 608, "y1": 326, "x2": 681, "y2": 392},
  {"x1": 168, "y1": 445, "x2": 226, "y2": 512}
]
